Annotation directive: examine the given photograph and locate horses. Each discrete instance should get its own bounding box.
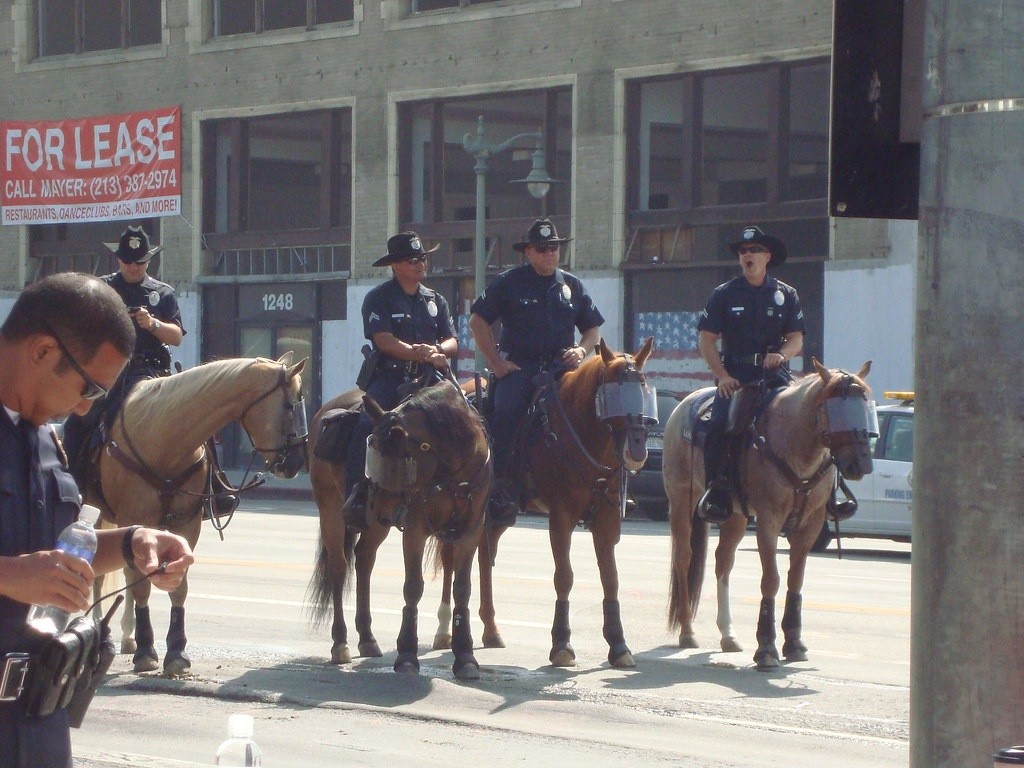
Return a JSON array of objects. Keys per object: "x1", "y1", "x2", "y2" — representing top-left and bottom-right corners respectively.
[
  {"x1": 306, "y1": 372, "x2": 485, "y2": 679},
  {"x1": 52, "y1": 348, "x2": 310, "y2": 672},
  {"x1": 667, "y1": 356, "x2": 879, "y2": 671},
  {"x1": 433, "y1": 335, "x2": 655, "y2": 668}
]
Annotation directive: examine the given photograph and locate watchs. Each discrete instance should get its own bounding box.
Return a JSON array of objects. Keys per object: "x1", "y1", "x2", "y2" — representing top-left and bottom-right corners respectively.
[{"x1": 148, "y1": 318, "x2": 161, "y2": 333}]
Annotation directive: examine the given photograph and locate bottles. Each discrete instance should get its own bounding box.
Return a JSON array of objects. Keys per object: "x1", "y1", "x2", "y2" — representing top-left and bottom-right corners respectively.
[{"x1": 26, "y1": 504, "x2": 100, "y2": 635}]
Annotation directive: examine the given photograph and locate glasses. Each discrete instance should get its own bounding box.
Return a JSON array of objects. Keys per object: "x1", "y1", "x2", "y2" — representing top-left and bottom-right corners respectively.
[
  {"x1": 398, "y1": 253, "x2": 429, "y2": 266},
  {"x1": 42, "y1": 319, "x2": 110, "y2": 402},
  {"x1": 122, "y1": 260, "x2": 147, "y2": 266},
  {"x1": 737, "y1": 245, "x2": 773, "y2": 255},
  {"x1": 532, "y1": 243, "x2": 559, "y2": 253}
]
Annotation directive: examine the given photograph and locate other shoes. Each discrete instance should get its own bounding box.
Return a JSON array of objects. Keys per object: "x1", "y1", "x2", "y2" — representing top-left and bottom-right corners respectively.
[
  {"x1": 344, "y1": 508, "x2": 370, "y2": 533},
  {"x1": 490, "y1": 499, "x2": 518, "y2": 520},
  {"x1": 203, "y1": 496, "x2": 236, "y2": 515},
  {"x1": 706, "y1": 501, "x2": 721, "y2": 518},
  {"x1": 625, "y1": 500, "x2": 636, "y2": 510},
  {"x1": 829, "y1": 499, "x2": 855, "y2": 517}
]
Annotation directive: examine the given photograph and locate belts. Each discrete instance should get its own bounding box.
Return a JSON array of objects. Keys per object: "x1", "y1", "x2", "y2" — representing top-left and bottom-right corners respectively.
[
  {"x1": 733, "y1": 353, "x2": 766, "y2": 366},
  {"x1": 386, "y1": 359, "x2": 424, "y2": 374}
]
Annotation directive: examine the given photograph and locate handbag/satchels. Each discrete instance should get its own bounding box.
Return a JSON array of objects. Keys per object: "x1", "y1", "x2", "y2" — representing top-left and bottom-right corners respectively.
[{"x1": 314, "y1": 408, "x2": 363, "y2": 463}]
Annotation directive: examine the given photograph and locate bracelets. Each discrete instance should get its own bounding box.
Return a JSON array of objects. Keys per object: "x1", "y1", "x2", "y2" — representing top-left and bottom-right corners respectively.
[
  {"x1": 120, "y1": 525, "x2": 144, "y2": 569},
  {"x1": 434, "y1": 344, "x2": 443, "y2": 353},
  {"x1": 577, "y1": 346, "x2": 586, "y2": 359},
  {"x1": 776, "y1": 352, "x2": 786, "y2": 363}
]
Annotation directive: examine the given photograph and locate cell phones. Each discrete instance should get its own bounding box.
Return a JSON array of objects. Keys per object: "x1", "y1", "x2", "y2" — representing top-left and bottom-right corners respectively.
[{"x1": 127, "y1": 307, "x2": 141, "y2": 314}]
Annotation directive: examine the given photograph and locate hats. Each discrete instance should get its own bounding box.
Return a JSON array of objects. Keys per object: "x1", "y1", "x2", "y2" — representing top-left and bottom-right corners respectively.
[
  {"x1": 730, "y1": 224, "x2": 789, "y2": 267},
  {"x1": 372, "y1": 230, "x2": 441, "y2": 267},
  {"x1": 102, "y1": 225, "x2": 167, "y2": 264},
  {"x1": 511, "y1": 219, "x2": 575, "y2": 252}
]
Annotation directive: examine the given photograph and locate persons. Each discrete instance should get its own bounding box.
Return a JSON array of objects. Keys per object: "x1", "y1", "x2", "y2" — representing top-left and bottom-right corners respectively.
[
  {"x1": 696, "y1": 225, "x2": 855, "y2": 519},
  {"x1": 0, "y1": 272, "x2": 193, "y2": 578},
  {"x1": 345, "y1": 230, "x2": 518, "y2": 533},
  {"x1": 62, "y1": 225, "x2": 236, "y2": 512},
  {"x1": 468, "y1": 219, "x2": 633, "y2": 523}
]
